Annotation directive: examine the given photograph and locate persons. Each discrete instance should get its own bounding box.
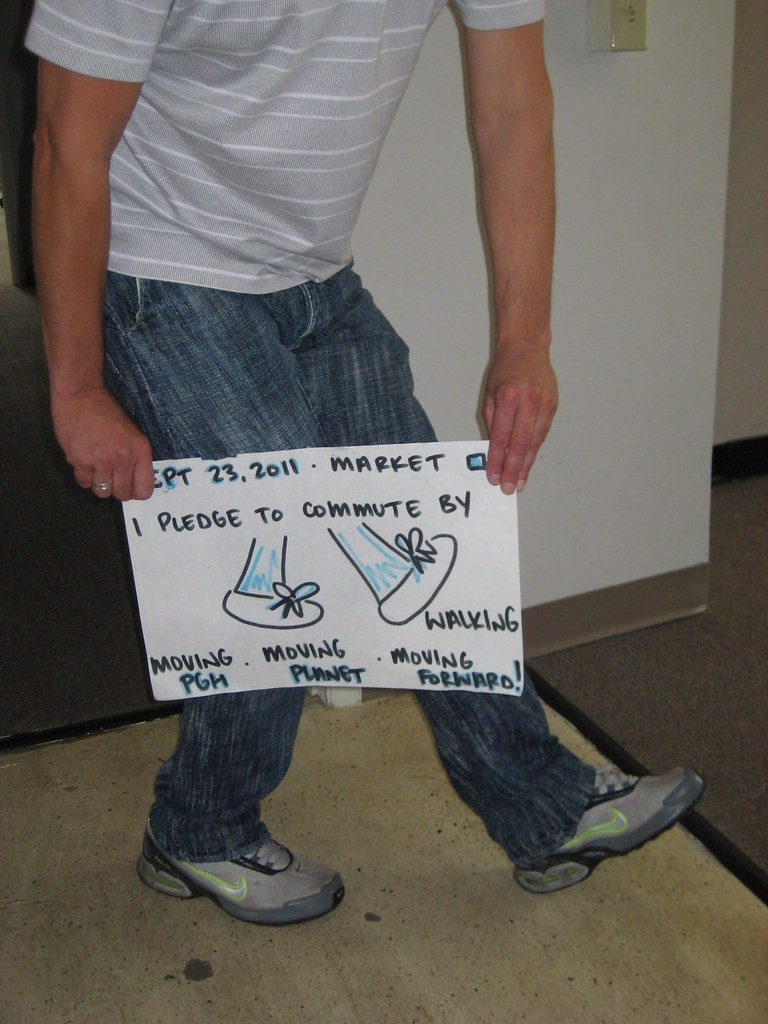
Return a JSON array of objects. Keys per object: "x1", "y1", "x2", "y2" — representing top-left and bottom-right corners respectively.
[{"x1": 23, "y1": 0, "x2": 705, "y2": 927}]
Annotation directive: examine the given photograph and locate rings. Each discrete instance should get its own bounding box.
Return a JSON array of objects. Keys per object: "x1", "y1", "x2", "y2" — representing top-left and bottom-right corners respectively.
[{"x1": 92, "y1": 479, "x2": 112, "y2": 490}]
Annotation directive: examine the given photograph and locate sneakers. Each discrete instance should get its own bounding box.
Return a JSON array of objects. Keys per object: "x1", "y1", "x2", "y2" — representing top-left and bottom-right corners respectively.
[
  {"x1": 513, "y1": 762, "x2": 704, "y2": 893},
  {"x1": 138, "y1": 816, "x2": 344, "y2": 925}
]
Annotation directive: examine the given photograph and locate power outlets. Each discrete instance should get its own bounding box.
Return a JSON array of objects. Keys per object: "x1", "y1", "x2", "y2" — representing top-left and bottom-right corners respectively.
[{"x1": 612, "y1": 0, "x2": 648, "y2": 50}]
